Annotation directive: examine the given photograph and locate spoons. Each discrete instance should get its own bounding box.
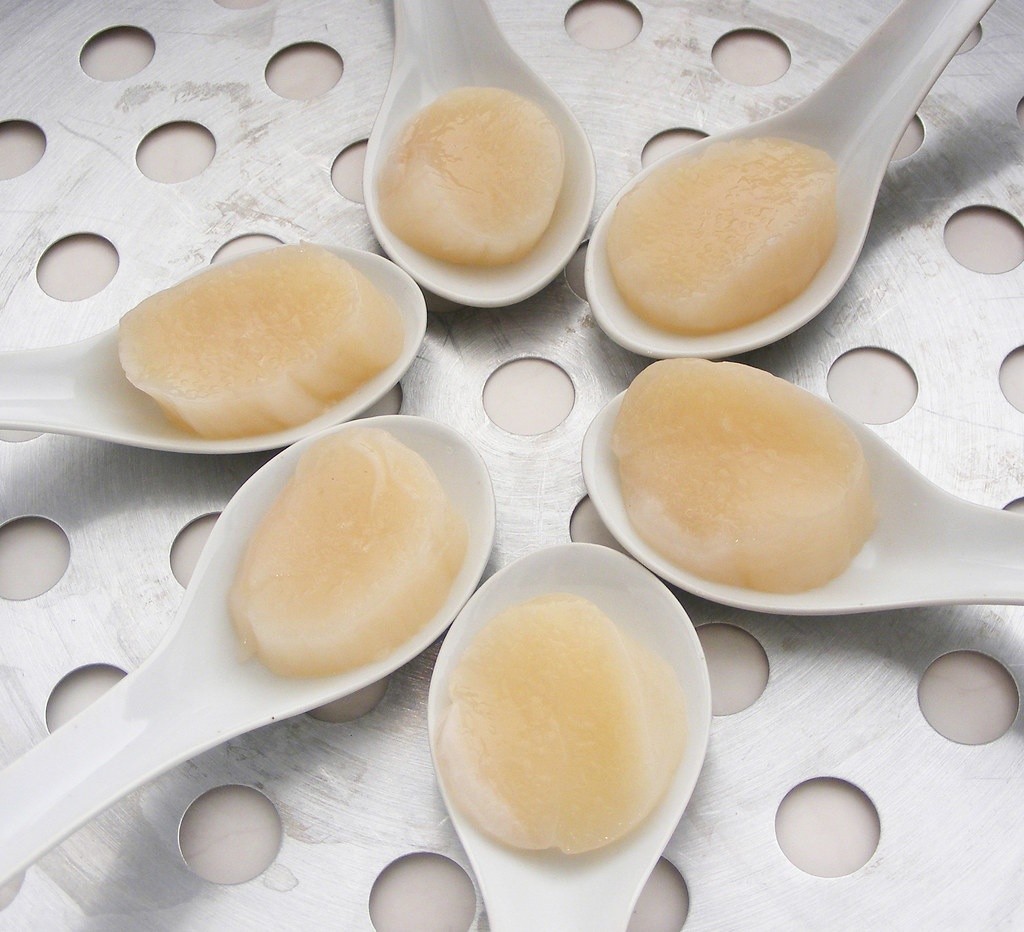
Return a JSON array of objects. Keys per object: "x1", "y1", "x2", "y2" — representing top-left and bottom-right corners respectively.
[
  {"x1": 584, "y1": 1, "x2": 998, "y2": 358},
  {"x1": 579, "y1": 363, "x2": 1024, "y2": 614},
  {"x1": 1, "y1": 249, "x2": 429, "y2": 452},
  {"x1": 359, "y1": 0, "x2": 594, "y2": 307},
  {"x1": 1, "y1": 415, "x2": 497, "y2": 896},
  {"x1": 426, "y1": 540, "x2": 714, "y2": 932}
]
